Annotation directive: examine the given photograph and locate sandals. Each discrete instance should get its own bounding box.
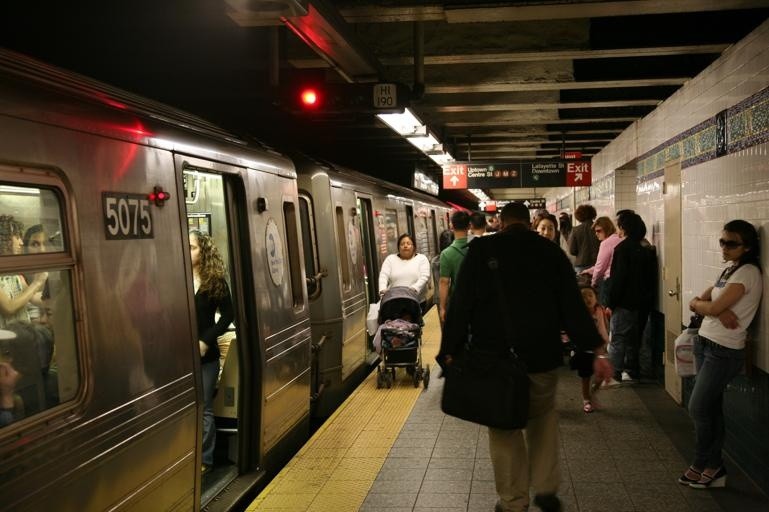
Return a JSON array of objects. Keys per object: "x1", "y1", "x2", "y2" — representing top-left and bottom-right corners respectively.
[
  {"x1": 678, "y1": 465, "x2": 701, "y2": 484},
  {"x1": 689, "y1": 465, "x2": 727, "y2": 489}
]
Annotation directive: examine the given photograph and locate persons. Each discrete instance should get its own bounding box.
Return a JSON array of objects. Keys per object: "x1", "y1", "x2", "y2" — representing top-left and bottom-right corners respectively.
[
  {"x1": 438, "y1": 201, "x2": 606, "y2": 511},
  {"x1": 678, "y1": 220, "x2": 764, "y2": 490},
  {"x1": 0, "y1": 216, "x2": 59, "y2": 427},
  {"x1": 189, "y1": 230, "x2": 234, "y2": 476},
  {"x1": 378, "y1": 201, "x2": 658, "y2": 412}
]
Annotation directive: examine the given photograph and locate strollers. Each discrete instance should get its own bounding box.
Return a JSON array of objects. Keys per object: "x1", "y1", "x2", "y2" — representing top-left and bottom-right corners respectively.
[{"x1": 374, "y1": 288, "x2": 433, "y2": 389}]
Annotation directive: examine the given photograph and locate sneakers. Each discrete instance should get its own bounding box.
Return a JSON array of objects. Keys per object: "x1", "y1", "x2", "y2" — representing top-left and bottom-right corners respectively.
[
  {"x1": 600, "y1": 377, "x2": 621, "y2": 390},
  {"x1": 583, "y1": 399, "x2": 593, "y2": 413},
  {"x1": 621, "y1": 372, "x2": 640, "y2": 384}
]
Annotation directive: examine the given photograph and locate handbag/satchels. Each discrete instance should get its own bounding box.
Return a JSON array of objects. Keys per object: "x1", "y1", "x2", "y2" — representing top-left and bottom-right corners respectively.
[
  {"x1": 442, "y1": 351, "x2": 529, "y2": 431},
  {"x1": 688, "y1": 268, "x2": 729, "y2": 334}
]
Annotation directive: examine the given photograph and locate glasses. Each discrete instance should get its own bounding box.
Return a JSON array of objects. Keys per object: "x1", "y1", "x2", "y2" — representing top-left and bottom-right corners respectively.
[{"x1": 718, "y1": 239, "x2": 741, "y2": 249}]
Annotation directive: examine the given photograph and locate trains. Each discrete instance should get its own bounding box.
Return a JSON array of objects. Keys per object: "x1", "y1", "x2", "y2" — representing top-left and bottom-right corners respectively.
[{"x1": 0, "y1": 49, "x2": 458, "y2": 512}]
